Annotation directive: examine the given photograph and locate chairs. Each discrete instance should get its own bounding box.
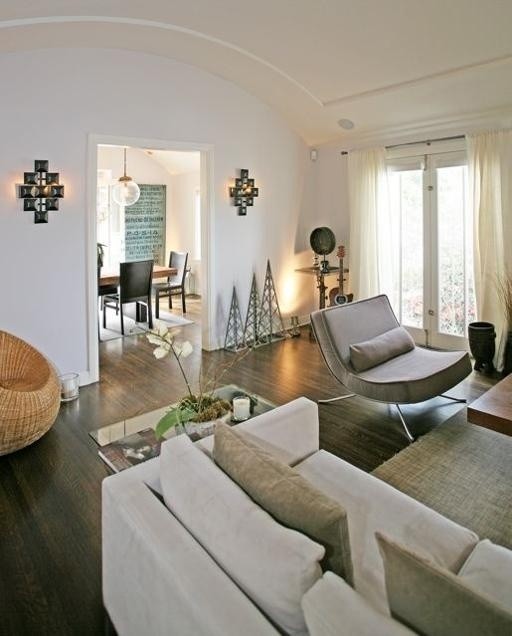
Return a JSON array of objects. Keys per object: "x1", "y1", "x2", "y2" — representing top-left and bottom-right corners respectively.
[
  {"x1": 151, "y1": 251, "x2": 189, "y2": 319},
  {"x1": 103, "y1": 260, "x2": 155, "y2": 336},
  {"x1": 1, "y1": 328, "x2": 61, "y2": 457}
]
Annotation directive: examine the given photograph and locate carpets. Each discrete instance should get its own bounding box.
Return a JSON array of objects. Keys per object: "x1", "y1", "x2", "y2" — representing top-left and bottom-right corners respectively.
[
  {"x1": 369, "y1": 405, "x2": 511, "y2": 550},
  {"x1": 98, "y1": 295, "x2": 195, "y2": 342}
]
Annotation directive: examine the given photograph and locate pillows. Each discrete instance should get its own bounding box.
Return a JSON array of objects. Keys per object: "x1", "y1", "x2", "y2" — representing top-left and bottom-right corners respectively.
[
  {"x1": 349, "y1": 326, "x2": 416, "y2": 372},
  {"x1": 375, "y1": 530, "x2": 512, "y2": 636},
  {"x1": 213, "y1": 419, "x2": 354, "y2": 588}
]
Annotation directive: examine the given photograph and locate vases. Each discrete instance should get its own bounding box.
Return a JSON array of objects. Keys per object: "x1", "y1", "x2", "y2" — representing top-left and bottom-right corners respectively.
[{"x1": 183, "y1": 411, "x2": 231, "y2": 442}]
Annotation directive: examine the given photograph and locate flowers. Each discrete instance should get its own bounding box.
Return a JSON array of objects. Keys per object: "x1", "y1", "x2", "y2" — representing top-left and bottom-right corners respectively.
[{"x1": 137, "y1": 323, "x2": 258, "y2": 441}]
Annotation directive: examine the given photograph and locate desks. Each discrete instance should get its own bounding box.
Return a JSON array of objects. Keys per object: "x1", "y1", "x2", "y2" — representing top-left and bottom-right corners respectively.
[
  {"x1": 468, "y1": 373, "x2": 512, "y2": 437},
  {"x1": 296, "y1": 264, "x2": 350, "y2": 309},
  {"x1": 99, "y1": 263, "x2": 178, "y2": 287}
]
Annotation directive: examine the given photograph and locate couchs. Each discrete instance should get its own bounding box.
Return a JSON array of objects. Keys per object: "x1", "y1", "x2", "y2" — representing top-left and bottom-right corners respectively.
[
  {"x1": 102, "y1": 397, "x2": 512, "y2": 635},
  {"x1": 309, "y1": 293, "x2": 474, "y2": 441}
]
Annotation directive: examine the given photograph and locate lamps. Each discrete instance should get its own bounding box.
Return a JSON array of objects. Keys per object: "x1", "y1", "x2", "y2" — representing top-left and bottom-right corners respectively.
[
  {"x1": 228, "y1": 168, "x2": 259, "y2": 215},
  {"x1": 112, "y1": 148, "x2": 140, "y2": 206},
  {"x1": 287, "y1": 316, "x2": 301, "y2": 336}
]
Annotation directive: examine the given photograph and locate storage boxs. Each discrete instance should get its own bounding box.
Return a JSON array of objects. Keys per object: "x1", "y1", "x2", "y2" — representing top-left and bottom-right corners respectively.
[{"x1": 16, "y1": 159, "x2": 64, "y2": 224}]
